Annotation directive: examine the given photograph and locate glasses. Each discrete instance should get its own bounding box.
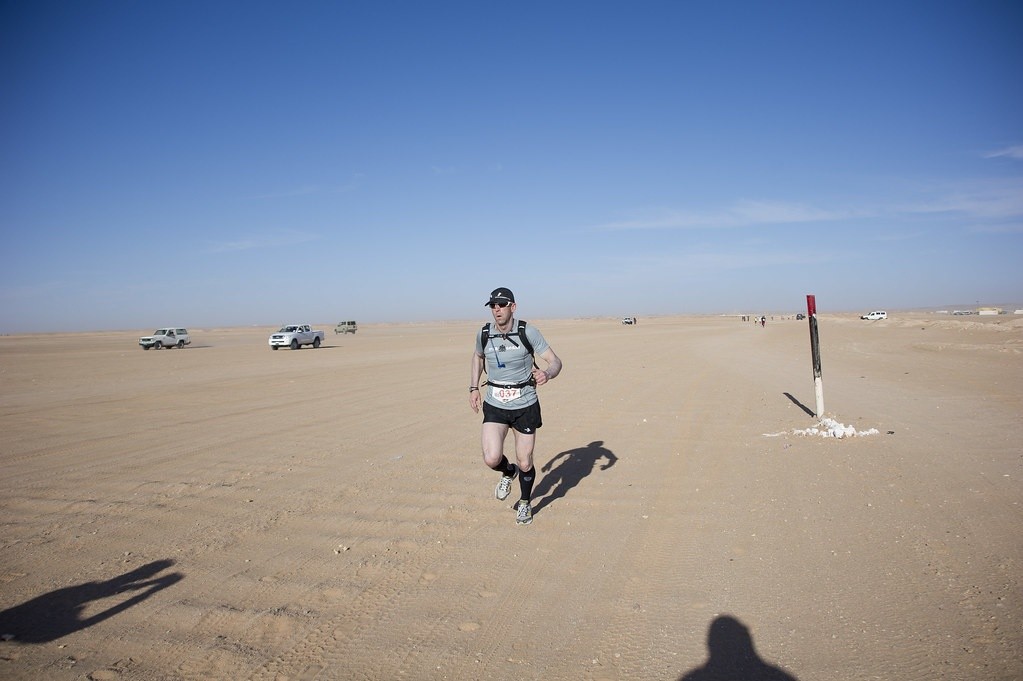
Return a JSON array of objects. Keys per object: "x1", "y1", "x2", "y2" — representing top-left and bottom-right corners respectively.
[{"x1": 490, "y1": 302, "x2": 513, "y2": 309}]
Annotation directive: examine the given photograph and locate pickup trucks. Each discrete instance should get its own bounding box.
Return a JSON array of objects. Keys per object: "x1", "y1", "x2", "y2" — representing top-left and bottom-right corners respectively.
[
  {"x1": 861, "y1": 311, "x2": 887, "y2": 320},
  {"x1": 138, "y1": 328, "x2": 191, "y2": 350},
  {"x1": 268, "y1": 324, "x2": 325, "y2": 350}
]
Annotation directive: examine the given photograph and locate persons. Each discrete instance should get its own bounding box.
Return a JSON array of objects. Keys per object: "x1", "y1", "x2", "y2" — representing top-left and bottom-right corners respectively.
[
  {"x1": 468, "y1": 287, "x2": 562, "y2": 524},
  {"x1": 742, "y1": 316, "x2": 790, "y2": 328}
]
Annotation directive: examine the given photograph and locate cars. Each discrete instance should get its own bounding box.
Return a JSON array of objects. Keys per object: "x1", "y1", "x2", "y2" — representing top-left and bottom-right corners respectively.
[
  {"x1": 796, "y1": 314, "x2": 802, "y2": 320},
  {"x1": 622, "y1": 317, "x2": 632, "y2": 325}
]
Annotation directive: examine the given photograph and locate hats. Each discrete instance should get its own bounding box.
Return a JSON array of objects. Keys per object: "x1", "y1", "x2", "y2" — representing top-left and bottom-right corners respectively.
[{"x1": 485, "y1": 288, "x2": 515, "y2": 306}]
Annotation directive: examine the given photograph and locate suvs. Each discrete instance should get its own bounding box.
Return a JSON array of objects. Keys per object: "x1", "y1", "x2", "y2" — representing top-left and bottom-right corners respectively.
[{"x1": 335, "y1": 320, "x2": 357, "y2": 334}]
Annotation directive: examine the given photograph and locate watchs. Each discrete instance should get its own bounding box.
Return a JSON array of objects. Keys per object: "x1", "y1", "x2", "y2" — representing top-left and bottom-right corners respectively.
[{"x1": 469, "y1": 387, "x2": 478, "y2": 392}]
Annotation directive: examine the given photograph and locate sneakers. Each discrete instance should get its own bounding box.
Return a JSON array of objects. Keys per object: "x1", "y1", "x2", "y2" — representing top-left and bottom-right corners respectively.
[
  {"x1": 495, "y1": 463, "x2": 519, "y2": 501},
  {"x1": 516, "y1": 499, "x2": 533, "y2": 524}
]
